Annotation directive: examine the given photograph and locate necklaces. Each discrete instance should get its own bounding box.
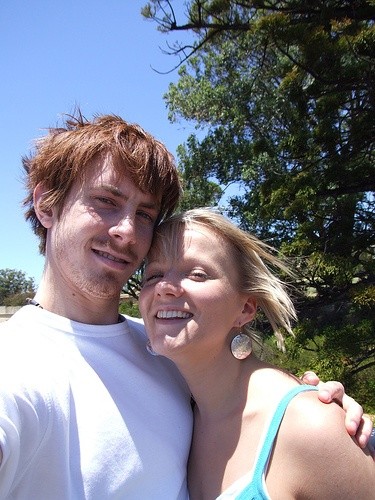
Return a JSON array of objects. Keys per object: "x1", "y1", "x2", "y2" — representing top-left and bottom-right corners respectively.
[{"x1": 24, "y1": 297, "x2": 45, "y2": 311}]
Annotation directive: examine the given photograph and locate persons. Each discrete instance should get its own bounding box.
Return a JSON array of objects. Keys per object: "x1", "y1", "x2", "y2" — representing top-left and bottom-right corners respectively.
[
  {"x1": 0, "y1": 102, "x2": 373, "y2": 500},
  {"x1": 137, "y1": 204, "x2": 373, "y2": 499}
]
